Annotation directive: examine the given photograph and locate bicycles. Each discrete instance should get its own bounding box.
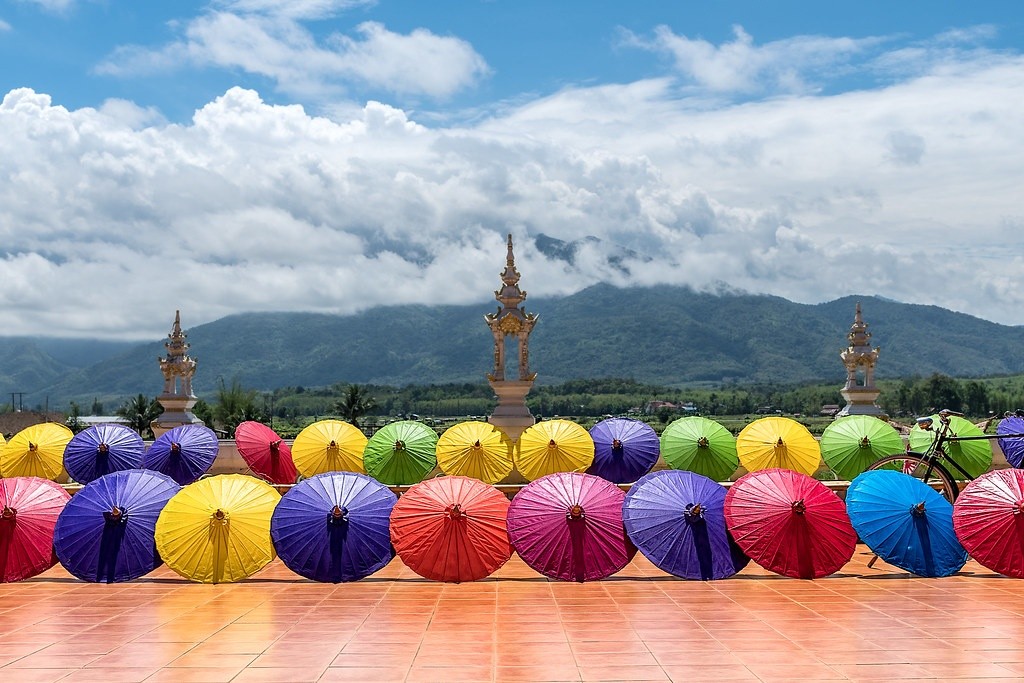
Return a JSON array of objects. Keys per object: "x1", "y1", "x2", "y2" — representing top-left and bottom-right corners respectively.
[{"x1": 863, "y1": 410, "x2": 1024, "y2": 541}]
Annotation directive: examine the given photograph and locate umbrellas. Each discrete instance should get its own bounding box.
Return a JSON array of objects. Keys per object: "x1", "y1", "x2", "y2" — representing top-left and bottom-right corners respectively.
[{"x1": 0, "y1": 412, "x2": 1024, "y2": 587}]
